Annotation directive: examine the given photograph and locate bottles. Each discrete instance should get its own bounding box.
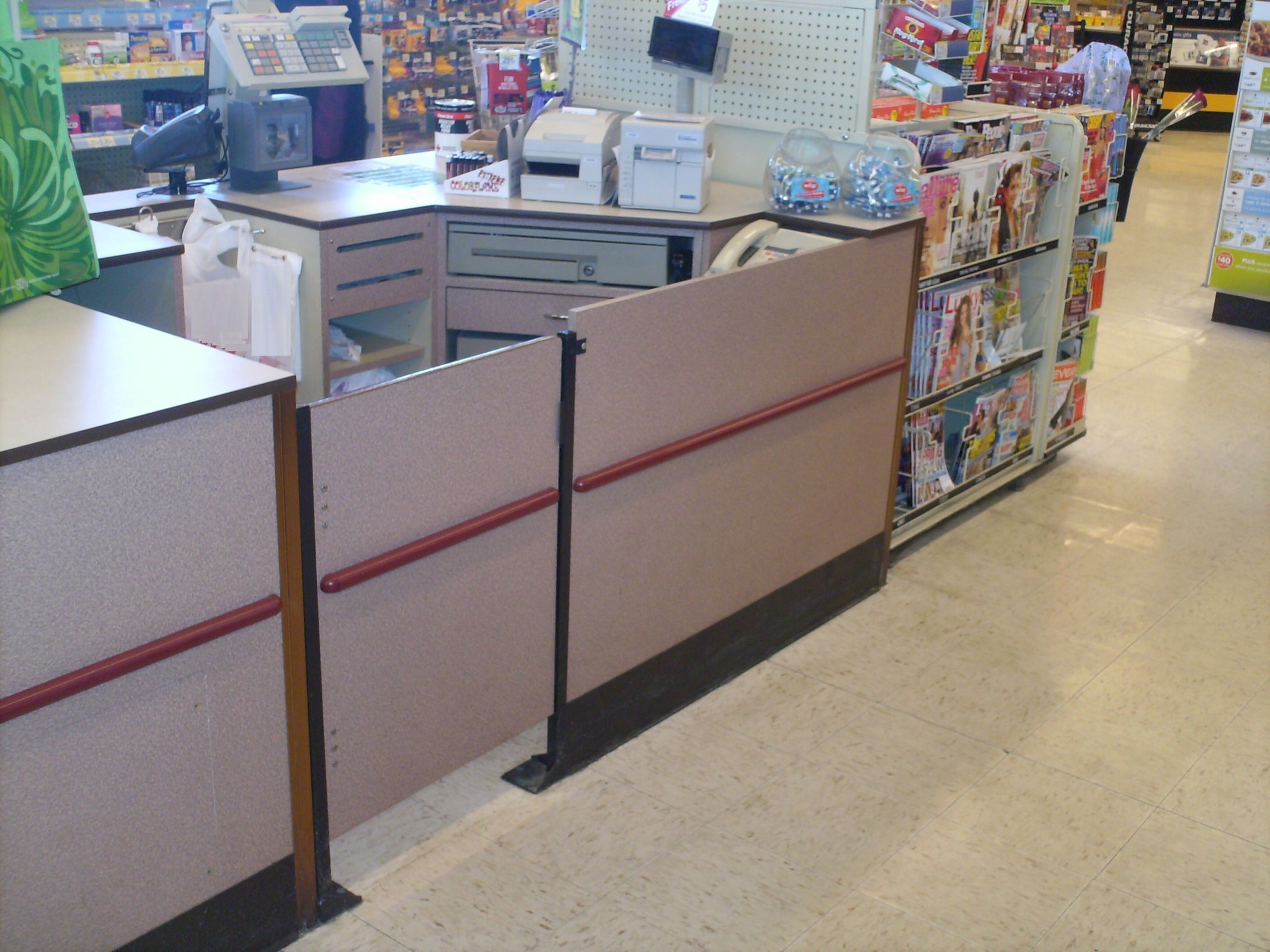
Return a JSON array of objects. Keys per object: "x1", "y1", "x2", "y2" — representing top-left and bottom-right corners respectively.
[
  {"x1": 142, "y1": 88, "x2": 202, "y2": 129},
  {"x1": 85, "y1": 41, "x2": 102, "y2": 65},
  {"x1": 841, "y1": 131, "x2": 922, "y2": 220},
  {"x1": 763, "y1": 125, "x2": 842, "y2": 215}
]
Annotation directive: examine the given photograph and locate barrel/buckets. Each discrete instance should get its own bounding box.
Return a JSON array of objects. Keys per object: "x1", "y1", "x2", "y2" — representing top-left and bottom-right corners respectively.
[{"x1": 434, "y1": 98, "x2": 476, "y2": 158}]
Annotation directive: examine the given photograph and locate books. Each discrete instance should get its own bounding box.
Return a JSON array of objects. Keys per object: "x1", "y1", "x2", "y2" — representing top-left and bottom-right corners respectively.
[{"x1": 888, "y1": 102, "x2": 1042, "y2": 521}]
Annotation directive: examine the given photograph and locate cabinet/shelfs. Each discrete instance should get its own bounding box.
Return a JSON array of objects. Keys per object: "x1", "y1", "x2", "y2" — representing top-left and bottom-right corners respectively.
[{"x1": 0, "y1": 0, "x2": 1247, "y2": 952}]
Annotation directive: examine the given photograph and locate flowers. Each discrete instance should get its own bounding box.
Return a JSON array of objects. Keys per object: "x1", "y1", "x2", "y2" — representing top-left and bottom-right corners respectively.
[
  {"x1": 1142, "y1": 90, "x2": 1207, "y2": 140},
  {"x1": 1124, "y1": 86, "x2": 1141, "y2": 137}
]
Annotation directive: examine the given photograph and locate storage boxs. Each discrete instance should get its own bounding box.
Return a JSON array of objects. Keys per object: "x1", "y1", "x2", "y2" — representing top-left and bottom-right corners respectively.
[
  {"x1": 1111, "y1": 15, "x2": 1122, "y2": 26},
  {"x1": 882, "y1": 61, "x2": 963, "y2": 107},
  {"x1": 171, "y1": 29, "x2": 207, "y2": 59},
  {"x1": 97, "y1": 41, "x2": 127, "y2": 63},
  {"x1": 1094, "y1": 16, "x2": 1111, "y2": 27},
  {"x1": 164, "y1": 19, "x2": 192, "y2": 31},
  {"x1": 915, "y1": 0, "x2": 972, "y2": 40},
  {"x1": 461, "y1": 129, "x2": 500, "y2": 162},
  {"x1": 887, "y1": 1, "x2": 959, "y2": 53},
  {"x1": 445, "y1": 118, "x2": 526, "y2": 199},
  {"x1": 872, "y1": 96, "x2": 949, "y2": 124},
  {"x1": 1075, "y1": 15, "x2": 1094, "y2": 25},
  {"x1": 115, "y1": 31, "x2": 151, "y2": 62}
]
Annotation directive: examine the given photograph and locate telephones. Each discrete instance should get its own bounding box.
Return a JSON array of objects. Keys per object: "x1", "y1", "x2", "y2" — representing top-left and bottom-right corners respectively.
[{"x1": 704, "y1": 219, "x2": 844, "y2": 276}]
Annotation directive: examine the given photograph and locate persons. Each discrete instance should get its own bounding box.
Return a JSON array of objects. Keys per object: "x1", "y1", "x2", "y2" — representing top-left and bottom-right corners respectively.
[
  {"x1": 936, "y1": 297, "x2": 981, "y2": 382},
  {"x1": 1020, "y1": 174, "x2": 1046, "y2": 247},
  {"x1": 1087, "y1": 115, "x2": 1111, "y2": 177},
  {"x1": 988, "y1": 164, "x2": 1021, "y2": 254},
  {"x1": 1208, "y1": 35, "x2": 1231, "y2": 69},
  {"x1": 970, "y1": 288, "x2": 994, "y2": 372},
  {"x1": 1027, "y1": 24, "x2": 1049, "y2": 46},
  {"x1": 919, "y1": 176, "x2": 960, "y2": 276},
  {"x1": 969, "y1": 408, "x2": 984, "y2": 442},
  {"x1": 990, "y1": 265, "x2": 1011, "y2": 310}
]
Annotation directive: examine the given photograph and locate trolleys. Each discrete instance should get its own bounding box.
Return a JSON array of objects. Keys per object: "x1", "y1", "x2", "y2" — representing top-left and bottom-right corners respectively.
[{"x1": 1129, "y1": 86, "x2": 1164, "y2": 142}]
